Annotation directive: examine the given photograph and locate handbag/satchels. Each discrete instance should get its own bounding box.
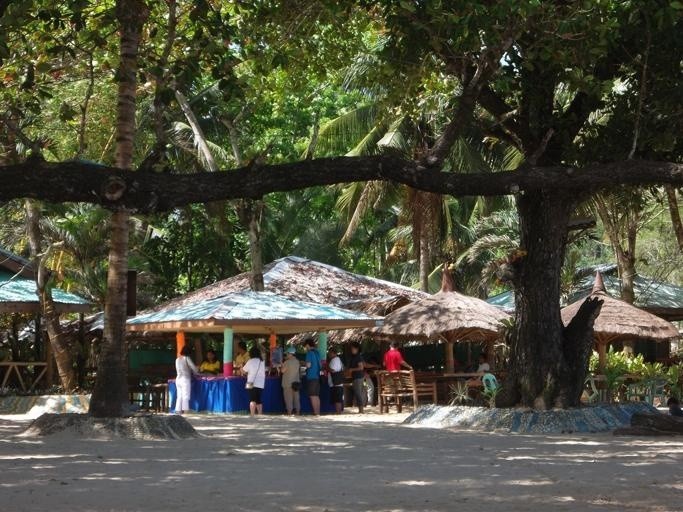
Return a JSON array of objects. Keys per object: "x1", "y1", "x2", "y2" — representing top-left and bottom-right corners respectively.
[
  {"x1": 320, "y1": 374, "x2": 326, "y2": 385},
  {"x1": 244, "y1": 381, "x2": 254, "y2": 390},
  {"x1": 330, "y1": 370, "x2": 346, "y2": 386},
  {"x1": 291, "y1": 381, "x2": 304, "y2": 391}
]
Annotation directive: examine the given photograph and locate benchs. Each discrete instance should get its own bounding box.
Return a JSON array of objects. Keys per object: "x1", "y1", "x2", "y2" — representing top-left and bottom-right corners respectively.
[{"x1": 375, "y1": 370, "x2": 437, "y2": 415}]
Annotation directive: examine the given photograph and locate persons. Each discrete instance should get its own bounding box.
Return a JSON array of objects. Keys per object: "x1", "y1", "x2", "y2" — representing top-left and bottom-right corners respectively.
[
  {"x1": 463, "y1": 354, "x2": 490, "y2": 396},
  {"x1": 175, "y1": 346, "x2": 200, "y2": 414},
  {"x1": 383, "y1": 341, "x2": 413, "y2": 403},
  {"x1": 665, "y1": 397, "x2": 683, "y2": 416},
  {"x1": 233, "y1": 341, "x2": 380, "y2": 418},
  {"x1": 199, "y1": 349, "x2": 222, "y2": 375}
]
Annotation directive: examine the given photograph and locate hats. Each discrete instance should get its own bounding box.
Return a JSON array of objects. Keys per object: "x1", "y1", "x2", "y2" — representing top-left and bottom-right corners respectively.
[{"x1": 283, "y1": 347, "x2": 296, "y2": 354}]
